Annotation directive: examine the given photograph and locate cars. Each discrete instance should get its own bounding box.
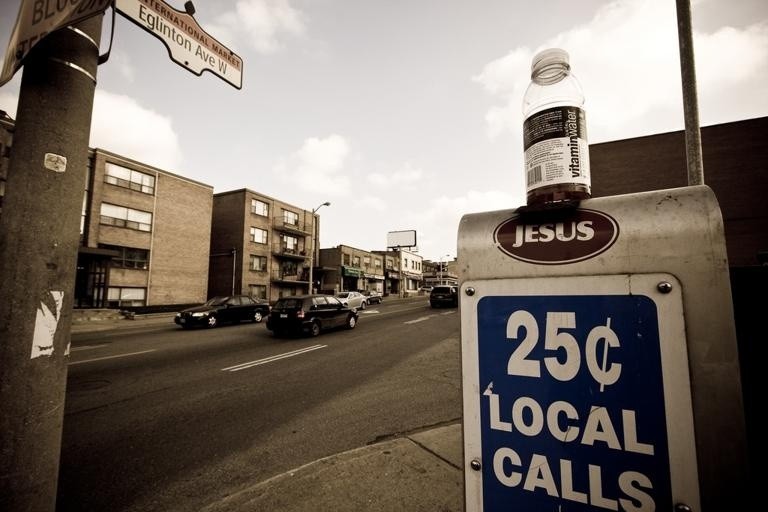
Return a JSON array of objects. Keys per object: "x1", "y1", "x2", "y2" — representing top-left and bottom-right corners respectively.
[
  {"x1": 429, "y1": 284, "x2": 458, "y2": 310},
  {"x1": 264, "y1": 293, "x2": 359, "y2": 338},
  {"x1": 360, "y1": 290, "x2": 384, "y2": 306},
  {"x1": 173, "y1": 294, "x2": 271, "y2": 330},
  {"x1": 334, "y1": 292, "x2": 368, "y2": 311}
]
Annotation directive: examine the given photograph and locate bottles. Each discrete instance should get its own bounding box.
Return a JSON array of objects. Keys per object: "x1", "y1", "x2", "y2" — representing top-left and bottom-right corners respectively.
[{"x1": 520, "y1": 43, "x2": 594, "y2": 204}]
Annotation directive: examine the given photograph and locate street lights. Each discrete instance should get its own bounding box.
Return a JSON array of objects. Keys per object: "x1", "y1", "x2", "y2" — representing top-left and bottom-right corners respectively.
[{"x1": 307, "y1": 201, "x2": 331, "y2": 294}]
